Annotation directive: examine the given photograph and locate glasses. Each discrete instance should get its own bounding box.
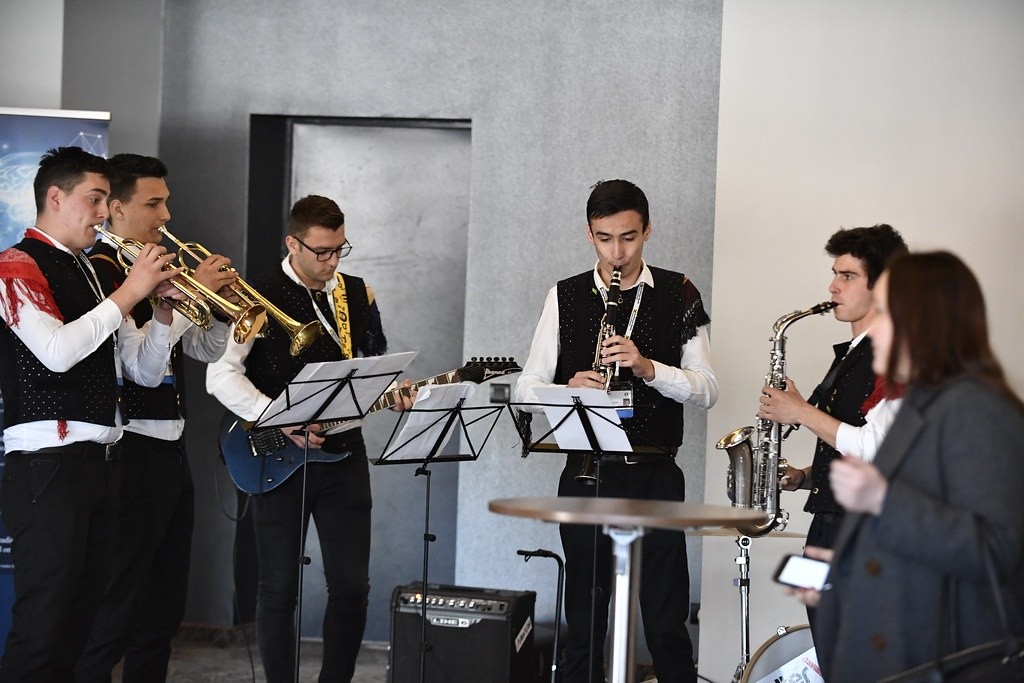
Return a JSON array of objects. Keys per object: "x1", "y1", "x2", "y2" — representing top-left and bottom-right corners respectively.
[{"x1": 293, "y1": 236, "x2": 353, "y2": 262}]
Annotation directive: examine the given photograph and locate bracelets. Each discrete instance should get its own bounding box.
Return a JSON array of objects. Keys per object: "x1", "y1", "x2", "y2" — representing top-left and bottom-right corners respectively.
[{"x1": 793, "y1": 469, "x2": 806, "y2": 492}]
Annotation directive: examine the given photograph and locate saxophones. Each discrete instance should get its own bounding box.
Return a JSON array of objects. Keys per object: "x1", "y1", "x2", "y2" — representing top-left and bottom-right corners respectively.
[{"x1": 716, "y1": 302, "x2": 843, "y2": 537}]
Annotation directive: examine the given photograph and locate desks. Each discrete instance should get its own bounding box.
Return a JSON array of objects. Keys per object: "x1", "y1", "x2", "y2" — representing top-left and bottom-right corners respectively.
[{"x1": 487, "y1": 497, "x2": 772, "y2": 683}]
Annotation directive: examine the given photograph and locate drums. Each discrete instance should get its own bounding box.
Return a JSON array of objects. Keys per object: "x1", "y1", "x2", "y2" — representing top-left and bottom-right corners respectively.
[{"x1": 739, "y1": 622, "x2": 826, "y2": 683}]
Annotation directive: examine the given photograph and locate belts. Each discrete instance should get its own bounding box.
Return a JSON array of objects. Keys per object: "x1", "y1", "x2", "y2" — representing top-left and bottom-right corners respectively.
[
  {"x1": 10, "y1": 441, "x2": 124, "y2": 461},
  {"x1": 567, "y1": 452, "x2": 672, "y2": 464}
]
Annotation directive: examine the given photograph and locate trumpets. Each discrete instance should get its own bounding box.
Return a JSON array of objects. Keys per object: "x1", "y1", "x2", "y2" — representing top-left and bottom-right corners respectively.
[{"x1": 92, "y1": 223, "x2": 321, "y2": 356}]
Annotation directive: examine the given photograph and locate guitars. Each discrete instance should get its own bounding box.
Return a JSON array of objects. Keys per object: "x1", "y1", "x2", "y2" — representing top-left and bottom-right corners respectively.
[{"x1": 218, "y1": 356, "x2": 523, "y2": 497}]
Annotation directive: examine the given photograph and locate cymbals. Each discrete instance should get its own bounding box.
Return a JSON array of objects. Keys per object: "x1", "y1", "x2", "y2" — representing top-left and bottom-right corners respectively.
[{"x1": 687, "y1": 528, "x2": 808, "y2": 538}]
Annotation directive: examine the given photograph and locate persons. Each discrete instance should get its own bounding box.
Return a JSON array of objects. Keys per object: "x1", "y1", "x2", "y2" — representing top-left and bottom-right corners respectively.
[
  {"x1": 624, "y1": 399, "x2": 629, "y2": 405},
  {"x1": 0, "y1": 142, "x2": 241, "y2": 682},
  {"x1": 512, "y1": 179, "x2": 721, "y2": 683},
  {"x1": 757, "y1": 224, "x2": 909, "y2": 681},
  {"x1": 203, "y1": 193, "x2": 419, "y2": 683},
  {"x1": 788, "y1": 251, "x2": 1024, "y2": 683}
]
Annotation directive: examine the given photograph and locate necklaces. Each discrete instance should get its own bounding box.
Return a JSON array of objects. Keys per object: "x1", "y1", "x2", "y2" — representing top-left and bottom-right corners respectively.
[{"x1": 598, "y1": 266, "x2": 642, "y2": 289}]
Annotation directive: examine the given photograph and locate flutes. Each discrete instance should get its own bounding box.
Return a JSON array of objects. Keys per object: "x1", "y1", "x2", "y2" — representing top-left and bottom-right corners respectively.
[{"x1": 573, "y1": 266, "x2": 622, "y2": 488}]
